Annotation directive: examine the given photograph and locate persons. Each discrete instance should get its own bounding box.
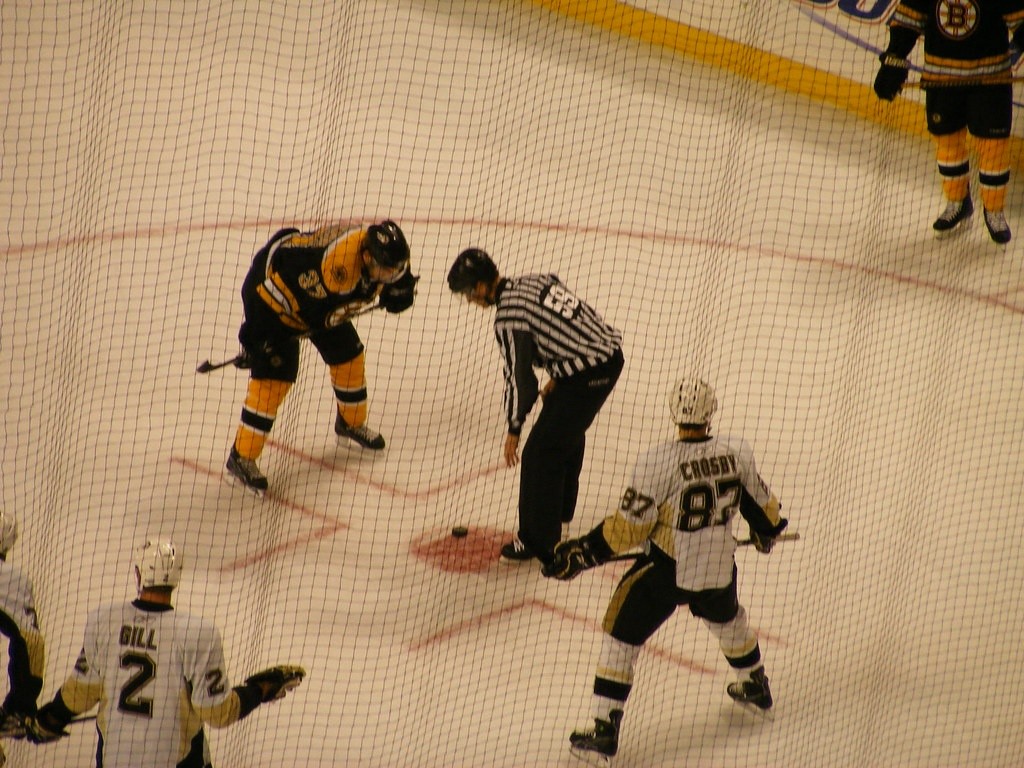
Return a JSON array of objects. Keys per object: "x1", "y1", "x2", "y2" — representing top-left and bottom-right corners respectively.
[
  {"x1": 448, "y1": 248, "x2": 624, "y2": 562},
  {"x1": 874, "y1": 0, "x2": 1024, "y2": 244},
  {"x1": 544, "y1": 382, "x2": 786, "y2": 756},
  {"x1": 0, "y1": 512, "x2": 44, "y2": 768},
  {"x1": 226, "y1": 222, "x2": 415, "y2": 491},
  {"x1": 26, "y1": 539, "x2": 304, "y2": 768}
]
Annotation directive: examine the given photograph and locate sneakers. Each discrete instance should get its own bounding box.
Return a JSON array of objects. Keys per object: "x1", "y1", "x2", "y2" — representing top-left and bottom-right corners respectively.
[
  {"x1": 933, "y1": 193, "x2": 974, "y2": 238},
  {"x1": 499, "y1": 538, "x2": 538, "y2": 564},
  {"x1": 727, "y1": 665, "x2": 775, "y2": 723},
  {"x1": 335, "y1": 402, "x2": 386, "y2": 456},
  {"x1": 225, "y1": 443, "x2": 268, "y2": 498},
  {"x1": 569, "y1": 709, "x2": 624, "y2": 768},
  {"x1": 983, "y1": 202, "x2": 1011, "y2": 251}
]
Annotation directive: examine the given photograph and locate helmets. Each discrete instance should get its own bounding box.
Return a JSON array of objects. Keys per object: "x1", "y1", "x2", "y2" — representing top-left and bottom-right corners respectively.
[
  {"x1": 447, "y1": 248, "x2": 497, "y2": 292},
  {"x1": 364, "y1": 220, "x2": 410, "y2": 270},
  {"x1": 0, "y1": 511, "x2": 18, "y2": 555},
  {"x1": 133, "y1": 537, "x2": 182, "y2": 586},
  {"x1": 669, "y1": 377, "x2": 718, "y2": 424}
]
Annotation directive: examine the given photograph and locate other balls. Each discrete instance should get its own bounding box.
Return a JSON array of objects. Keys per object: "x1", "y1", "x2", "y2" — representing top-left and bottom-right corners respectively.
[{"x1": 452, "y1": 526, "x2": 467, "y2": 537}]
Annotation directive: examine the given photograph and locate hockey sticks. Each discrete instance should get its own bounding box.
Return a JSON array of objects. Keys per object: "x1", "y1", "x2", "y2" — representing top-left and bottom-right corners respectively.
[
  {"x1": 0, "y1": 714, "x2": 98, "y2": 739},
  {"x1": 788, "y1": 0, "x2": 925, "y2": 74},
  {"x1": 532, "y1": 532, "x2": 802, "y2": 573},
  {"x1": 195, "y1": 272, "x2": 421, "y2": 374}
]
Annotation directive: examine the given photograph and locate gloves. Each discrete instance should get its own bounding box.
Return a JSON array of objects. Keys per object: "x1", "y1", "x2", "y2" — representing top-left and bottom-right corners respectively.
[
  {"x1": 749, "y1": 504, "x2": 788, "y2": 553},
  {"x1": 246, "y1": 664, "x2": 306, "y2": 703},
  {"x1": 0, "y1": 690, "x2": 37, "y2": 740},
  {"x1": 31, "y1": 701, "x2": 70, "y2": 743},
  {"x1": 873, "y1": 53, "x2": 911, "y2": 102},
  {"x1": 235, "y1": 347, "x2": 287, "y2": 369},
  {"x1": 542, "y1": 521, "x2": 614, "y2": 580},
  {"x1": 379, "y1": 271, "x2": 415, "y2": 314}
]
